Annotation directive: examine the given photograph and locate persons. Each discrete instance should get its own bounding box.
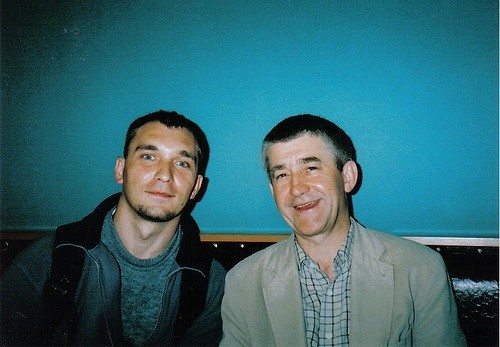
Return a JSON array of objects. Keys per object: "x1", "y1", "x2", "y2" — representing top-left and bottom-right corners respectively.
[
  {"x1": 218, "y1": 113, "x2": 467, "y2": 347},
  {"x1": 0, "y1": 109, "x2": 227, "y2": 347}
]
